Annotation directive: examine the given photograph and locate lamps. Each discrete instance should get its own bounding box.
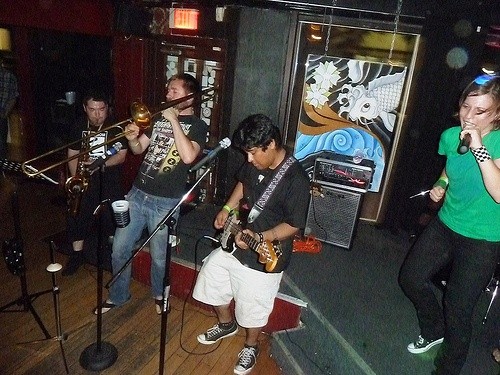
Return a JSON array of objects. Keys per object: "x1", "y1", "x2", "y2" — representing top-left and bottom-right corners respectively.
[{"x1": 168, "y1": 2, "x2": 198, "y2": 30}]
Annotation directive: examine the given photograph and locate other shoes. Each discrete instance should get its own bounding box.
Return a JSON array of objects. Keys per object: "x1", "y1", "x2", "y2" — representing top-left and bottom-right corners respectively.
[{"x1": 61, "y1": 262, "x2": 77, "y2": 276}]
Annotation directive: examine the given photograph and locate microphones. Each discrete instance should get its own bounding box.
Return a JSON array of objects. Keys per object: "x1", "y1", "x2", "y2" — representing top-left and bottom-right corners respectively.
[
  {"x1": 188, "y1": 137, "x2": 231, "y2": 175},
  {"x1": 89, "y1": 142, "x2": 122, "y2": 171},
  {"x1": 459, "y1": 134, "x2": 471, "y2": 154}
]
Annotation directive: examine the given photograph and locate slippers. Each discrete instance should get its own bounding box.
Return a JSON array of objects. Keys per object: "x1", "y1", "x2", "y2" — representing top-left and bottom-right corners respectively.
[
  {"x1": 92, "y1": 293, "x2": 133, "y2": 315},
  {"x1": 155, "y1": 297, "x2": 172, "y2": 316}
]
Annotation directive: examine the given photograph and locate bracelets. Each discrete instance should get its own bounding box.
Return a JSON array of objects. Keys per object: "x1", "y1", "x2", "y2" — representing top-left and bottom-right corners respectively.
[
  {"x1": 470, "y1": 144, "x2": 491, "y2": 163},
  {"x1": 433, "y1": 176, "x2": 448, "y2": 189},
  {"x1": 256, "y1": 232, "x2": 263, "y2": 243},
  {"x1": 223, "y1": 204, "x2": 231, "y2": 212}
]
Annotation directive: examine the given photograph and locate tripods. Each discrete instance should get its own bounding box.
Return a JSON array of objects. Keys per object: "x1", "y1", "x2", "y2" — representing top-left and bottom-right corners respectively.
[{"x1": 0, "y1": 159, "x2": 93, "y2": 375}]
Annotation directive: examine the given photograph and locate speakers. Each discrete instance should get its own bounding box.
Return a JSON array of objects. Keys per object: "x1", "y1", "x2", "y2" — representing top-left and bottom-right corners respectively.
[{"x1": 301, "y1": 183, "x2": 364, "y2": 251}]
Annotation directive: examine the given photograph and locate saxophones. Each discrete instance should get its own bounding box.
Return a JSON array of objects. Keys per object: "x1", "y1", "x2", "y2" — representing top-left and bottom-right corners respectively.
[{"x1": 65, "y1": 119, "x2": 99, "y2": 218}]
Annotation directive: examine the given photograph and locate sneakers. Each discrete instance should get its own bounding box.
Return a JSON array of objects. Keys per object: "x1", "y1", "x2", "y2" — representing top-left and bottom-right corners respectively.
[
  {"x1": 197, "y1": 320, "x2": 239, "y2": 345},
  {"x1": 407, "y1": 335, "x2": 444, "y2": 354},
  {"x1": 234, "y1": 347, "x2": 259, "y2": 375}
]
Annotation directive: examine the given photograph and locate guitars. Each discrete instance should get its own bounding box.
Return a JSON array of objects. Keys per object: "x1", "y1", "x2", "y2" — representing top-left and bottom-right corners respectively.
[{"x1": 212, "y1": 211, "x2": 284, "y2": 272}]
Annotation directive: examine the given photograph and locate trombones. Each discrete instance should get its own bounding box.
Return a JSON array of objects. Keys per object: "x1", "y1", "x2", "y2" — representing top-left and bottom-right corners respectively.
[{"x1": 21, "y1": 87, "x2": 218, "y2": 176}]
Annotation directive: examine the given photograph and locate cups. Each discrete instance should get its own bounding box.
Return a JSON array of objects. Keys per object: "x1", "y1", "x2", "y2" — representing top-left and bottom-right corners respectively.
[
  {"x1": 353, "y1": 148, "x2": 363, "y2": 164},
  {"x1": 65, "y1": 91, "x2": 76, "y2": 105},
  {"x1": 112, "y1": 199, "x2": 129, "y2": 225}
]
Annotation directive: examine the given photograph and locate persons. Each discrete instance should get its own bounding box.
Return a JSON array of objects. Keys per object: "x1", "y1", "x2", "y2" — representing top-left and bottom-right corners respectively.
[
  {"x1": 94, "y1": 73, "x2": 208, "y2": 315},
  {"x1": 61, "y1": 88, "x2": 129, "y2": 278},
  {"x1": 398, "y1": 68, "x2": 500, "y2": 375},
  {"x1": 192, "y1": 113, "x2": 310, "y2": 375}
]
